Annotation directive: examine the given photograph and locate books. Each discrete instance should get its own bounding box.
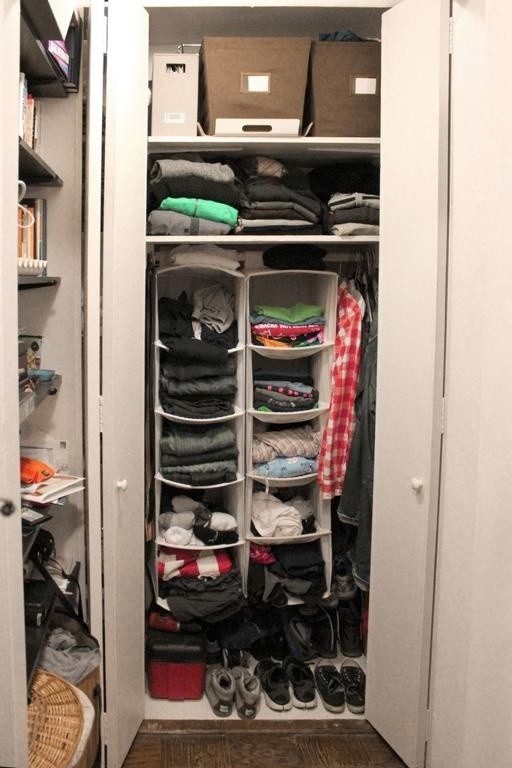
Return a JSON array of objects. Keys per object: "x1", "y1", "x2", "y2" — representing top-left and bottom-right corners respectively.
[
  {"x1": 17, "y1": 197, "x2": 48, "y2": 277},
  {"x1": 19, "y1": 69, "x2": 42, "y2": 154}
]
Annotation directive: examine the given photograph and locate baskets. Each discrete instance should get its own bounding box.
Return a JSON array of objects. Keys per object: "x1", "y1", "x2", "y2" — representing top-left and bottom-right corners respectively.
[{"x1": 27, "y1": 669, "x2": 89, "y2": 767}]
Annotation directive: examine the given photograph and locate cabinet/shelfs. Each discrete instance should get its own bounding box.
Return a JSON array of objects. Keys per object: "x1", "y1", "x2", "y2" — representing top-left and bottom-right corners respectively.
[{"x1": 0, "y1": 0, "x2": 452, "y2": 768}]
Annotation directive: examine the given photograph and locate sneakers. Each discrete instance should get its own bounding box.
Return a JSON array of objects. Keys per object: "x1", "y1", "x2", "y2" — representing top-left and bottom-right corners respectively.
[
  {"x1": 205, "y1": 601, "x2": 365, "y2": 720},
  {"x1": 323, "y1": 551, "x2": 357, "y2": 607}
]
